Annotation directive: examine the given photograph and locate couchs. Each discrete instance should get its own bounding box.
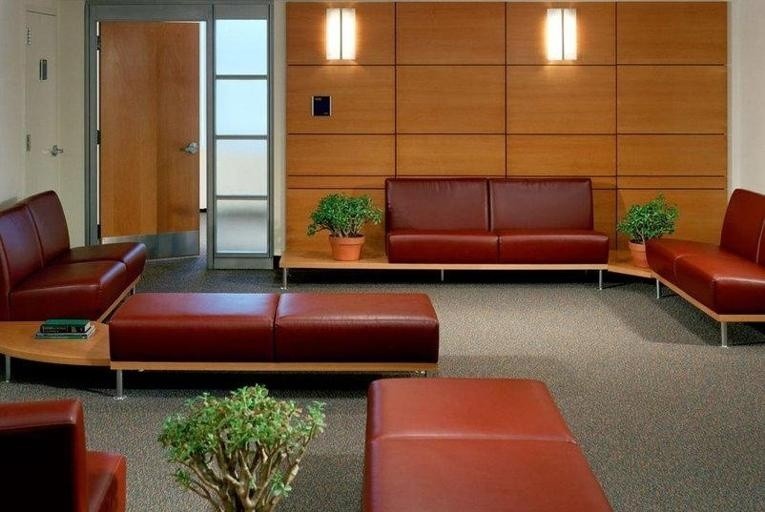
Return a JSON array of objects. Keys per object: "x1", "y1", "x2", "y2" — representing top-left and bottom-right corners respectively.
[
  {"x1": 0, "y1": 190, "x2": 146, "y2": 323},
  {"x1": 644, "y1": 188, "x2": 765, "y2": 348},
  {"x1": 1, "y1": 398, "x2": 127, "y2": 512},
  {"x1": 385, "y1": 177, "x2": 609, "y2": 292}
]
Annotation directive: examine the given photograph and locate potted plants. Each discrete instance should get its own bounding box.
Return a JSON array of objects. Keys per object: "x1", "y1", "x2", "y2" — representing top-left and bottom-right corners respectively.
[
  {"x1": 616, "y1": 192, "x2": 677, "y2": 268},
  {"x1": 307, "y1": 191, "x2": 384, "y2": 261}
]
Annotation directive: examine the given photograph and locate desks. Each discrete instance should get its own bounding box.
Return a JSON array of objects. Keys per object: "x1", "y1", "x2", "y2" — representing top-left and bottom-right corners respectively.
[{"x1": 0, "y1": 319, "x2": 113, "y2": 383}]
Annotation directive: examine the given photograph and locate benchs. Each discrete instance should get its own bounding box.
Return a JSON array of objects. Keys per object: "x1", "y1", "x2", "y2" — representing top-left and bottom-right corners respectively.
[
  {"x1": 108, "y1": 292, "x2": 439, "y2": 402},
  {"x1": 364, "y1": 379, "x2": 614, "y2": 512}
]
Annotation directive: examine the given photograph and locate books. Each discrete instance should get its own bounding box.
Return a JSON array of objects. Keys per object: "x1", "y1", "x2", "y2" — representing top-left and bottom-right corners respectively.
[{"x1": 34, "y1": 319, "x2": 97, "y2": 339}]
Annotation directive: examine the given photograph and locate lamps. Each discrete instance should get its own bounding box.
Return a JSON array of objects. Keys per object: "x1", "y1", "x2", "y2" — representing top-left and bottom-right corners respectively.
[
  {"x1": 546, "y1": 6, "x2": 577, "y2": 61},
  {"x1": 326, "y1": 6, "x2": 355, "y2": 61}
]
